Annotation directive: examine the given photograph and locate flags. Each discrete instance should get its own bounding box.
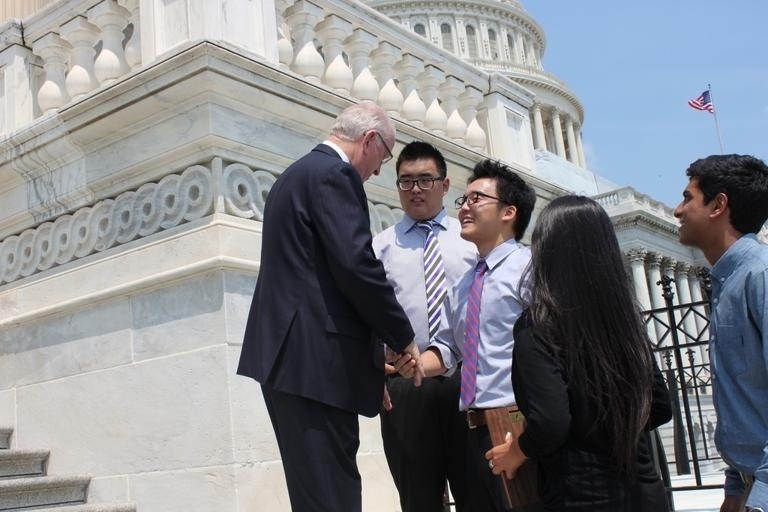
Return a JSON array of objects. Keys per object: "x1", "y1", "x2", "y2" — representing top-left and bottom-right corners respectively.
[{"x1": 688, "y1": 91, "x2": 713, "y2": 112}]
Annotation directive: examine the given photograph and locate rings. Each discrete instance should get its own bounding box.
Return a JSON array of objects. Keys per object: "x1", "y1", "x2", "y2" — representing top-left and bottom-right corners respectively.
[{"x1": 490, "y1": 459, "x2": 494, "y2": 467}]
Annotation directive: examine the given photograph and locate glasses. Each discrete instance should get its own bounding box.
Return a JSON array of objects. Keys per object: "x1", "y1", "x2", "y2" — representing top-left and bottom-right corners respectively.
[
  {"x1": 454, "y1": 192, "x2": 511, "y2": 210},
  {"x1": 362, "y1": 129, "x2": 393, "y2": 164},
  {"x1": 396, "y1": 176, "x2": 444, "y2": 191}
]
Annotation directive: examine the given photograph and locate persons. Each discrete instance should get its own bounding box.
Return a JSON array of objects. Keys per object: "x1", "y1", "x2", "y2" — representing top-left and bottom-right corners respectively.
[
  {"x1": 237, "y1": 101, "x2": 426, "y2": 512},
  {"x1": 393, "y1": 155, "x2": 533, "y2": 511},
  {"x1": 371, "y1": 141, "x2": 512, "y2": 511},
  {"x1": 486, "y1": 193, "x2": 673, "y2": 510},
  {"x1": 674, "y1": 153, "x2": 768, "y2": 512}
]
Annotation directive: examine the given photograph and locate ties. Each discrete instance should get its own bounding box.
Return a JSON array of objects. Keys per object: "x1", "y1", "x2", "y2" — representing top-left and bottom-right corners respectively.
[
  {"x1": 458, "y1": 260, "x2": 488, "y2": 412},
  {"x1": 418, "y1": 220, "x2": 449, "y2": 343}
]
